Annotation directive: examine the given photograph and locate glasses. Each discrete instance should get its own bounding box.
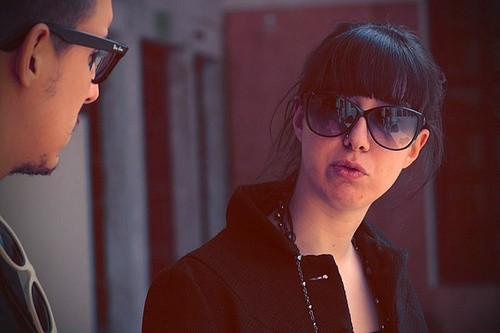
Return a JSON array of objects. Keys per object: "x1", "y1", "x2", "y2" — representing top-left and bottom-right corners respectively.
[
  {"x1": 38, "y1": 20, "x2": 129, "y2": 85},
  {"x1": 0, "y1": 216, "x2": 57, "y2": 332},
  {"x1": 304, "y1": 88, "x2": 425, "y2": 150}
]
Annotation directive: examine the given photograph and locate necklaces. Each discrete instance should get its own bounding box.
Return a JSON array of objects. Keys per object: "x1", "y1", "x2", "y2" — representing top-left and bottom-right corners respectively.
[{"x1": 274, "y1": 196, "x2": 389, "y2": 333}]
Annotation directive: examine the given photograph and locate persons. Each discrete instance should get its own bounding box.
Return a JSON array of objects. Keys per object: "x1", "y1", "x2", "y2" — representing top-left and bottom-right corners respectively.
[
  {"x1": 142, "y1": 21, "x2": 445, "y2": 333},
  {"x1": 0, "y1": 0, "x2": 130, "y2": 333}
]
